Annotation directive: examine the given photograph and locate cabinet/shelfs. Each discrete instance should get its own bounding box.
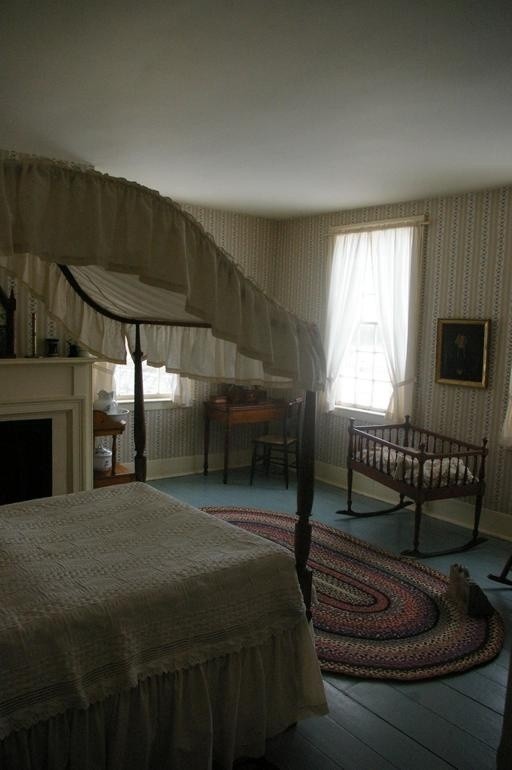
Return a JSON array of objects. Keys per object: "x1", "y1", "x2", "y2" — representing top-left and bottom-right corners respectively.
[{"x1": 93, "y1": 409, "x2": 136, "y2": 488}]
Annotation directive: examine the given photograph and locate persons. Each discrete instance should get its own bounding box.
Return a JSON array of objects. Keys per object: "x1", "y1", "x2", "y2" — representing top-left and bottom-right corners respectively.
[{"x1": 442, "y1": 334, "x2": 480, "y2": 382}]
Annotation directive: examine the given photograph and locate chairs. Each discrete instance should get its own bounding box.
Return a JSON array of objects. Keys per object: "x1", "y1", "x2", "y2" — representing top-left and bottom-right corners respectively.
[{"x1": 249, "y1": 398, "x2": 304, "y2": 490}]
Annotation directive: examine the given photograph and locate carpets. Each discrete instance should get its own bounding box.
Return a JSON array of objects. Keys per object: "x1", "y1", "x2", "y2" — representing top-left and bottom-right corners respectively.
[{"x1": 199, "y1": 505, "x2": 506, "y2": 682}]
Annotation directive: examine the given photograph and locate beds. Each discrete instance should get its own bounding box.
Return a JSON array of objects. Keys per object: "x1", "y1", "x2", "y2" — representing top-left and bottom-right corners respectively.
[
  {"x1": 334, "y1": 413, "x2": 489, "y2": 559},
  {"x1": 2, "y1": 480, "x2": 312, "y2": 770}
]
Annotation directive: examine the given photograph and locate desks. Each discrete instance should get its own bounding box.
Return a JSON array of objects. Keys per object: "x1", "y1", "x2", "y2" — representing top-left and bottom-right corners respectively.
[{"x1": 204, "y1": 399, "x2": 289, "y2": 486}]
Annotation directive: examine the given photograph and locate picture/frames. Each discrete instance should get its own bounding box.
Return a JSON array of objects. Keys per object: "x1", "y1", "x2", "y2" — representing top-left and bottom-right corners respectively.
[{"x1": 433, "y1": 317, "x2": 490, "y2": 389}]
